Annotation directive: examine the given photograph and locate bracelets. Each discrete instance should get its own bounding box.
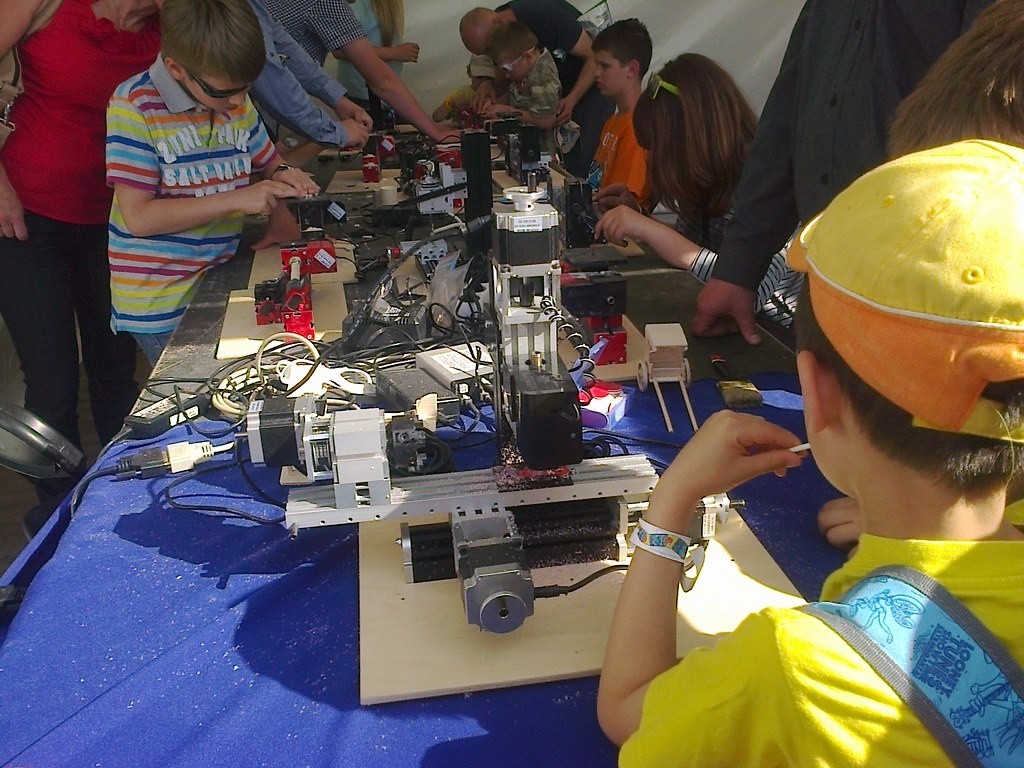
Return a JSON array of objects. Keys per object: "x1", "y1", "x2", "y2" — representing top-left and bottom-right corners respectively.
[
  {"x1": 629, "y1": 517, "x2": 692, "y2": 564},
  {"x1": 271, "y1": 162, "x2": 292, "y2": 179}
]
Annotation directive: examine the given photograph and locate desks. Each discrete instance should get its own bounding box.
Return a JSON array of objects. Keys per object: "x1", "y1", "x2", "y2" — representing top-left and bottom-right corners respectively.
[{"x1": 0, "y1": 115, "x2": 863, "y2": 767}]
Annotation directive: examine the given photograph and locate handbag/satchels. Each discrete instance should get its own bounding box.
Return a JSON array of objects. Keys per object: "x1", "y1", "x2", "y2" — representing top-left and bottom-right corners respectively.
[{"x1": 0, "y1": 0, "x2": 63, "y2": 148}]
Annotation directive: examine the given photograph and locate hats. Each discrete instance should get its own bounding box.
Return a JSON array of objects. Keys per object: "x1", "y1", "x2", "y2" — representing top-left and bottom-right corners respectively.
[{"x1": 785, "y1": 140, "x2": 1024, "y2": 443}]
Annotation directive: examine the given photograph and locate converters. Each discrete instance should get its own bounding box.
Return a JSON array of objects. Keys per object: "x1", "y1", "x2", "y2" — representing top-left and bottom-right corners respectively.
[{"x1": 125, "y1": 390, "x2": 207, "y2": 440}]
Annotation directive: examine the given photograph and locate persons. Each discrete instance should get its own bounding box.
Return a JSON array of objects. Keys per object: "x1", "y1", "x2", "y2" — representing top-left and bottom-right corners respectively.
[
  {"x1": 429, "y1": 0, "x2": 617, "y2": 180},
  {"x1": 590, "y1": 52, "x2": 806, "y2": 330},
  {"x1": 330, "y1": 0, "x2": 421, "y2": 131},
  {"x1": 245, "y1": 0, "x2": 374, "y2": 150},
  {"x1": 693, "y1": 0, "x2": 996, "y2": 345},
  {"x1": 0, "y1": 0, "x2": 322, "y2": 462},
  {"x1": 585, "y1": 17, "x2": 652, "y2": 213},
  {"x1": 107, "y1": 0, "x2": 323, "y2": 368},
  {"x1": 812, "y1": 1, "x2": 1024, "y2": 561},
  {"x1": 595, "y1": 139, "x2": 1024, "y2": 768},
  {"x1": 250, "y1": 0, "x2": 471, "y2": 143}
]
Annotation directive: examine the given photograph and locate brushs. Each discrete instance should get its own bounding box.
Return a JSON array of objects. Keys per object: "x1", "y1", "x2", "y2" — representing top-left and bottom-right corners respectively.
[{"x1": 710, "y1": 353, "x2": 764, "y2": 410}]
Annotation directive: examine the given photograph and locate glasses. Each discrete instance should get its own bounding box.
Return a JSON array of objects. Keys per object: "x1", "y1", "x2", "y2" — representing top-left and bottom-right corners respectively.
[
  {"x1": 185, "y1": 66, "x2": 252, "y2": 98},
  {"x1": 647, "y1": 71, "x2": 681, "y2": 101},
  {"x1": 496, "y1": 46, "x2": 535, "y2": 73}
]
